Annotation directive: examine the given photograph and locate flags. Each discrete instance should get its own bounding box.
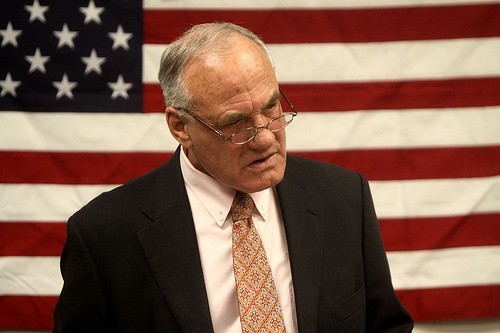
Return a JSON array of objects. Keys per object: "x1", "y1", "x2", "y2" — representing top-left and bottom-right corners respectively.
[{"x1": 0, "y1": 0, "x2": 500, "y2": 333}]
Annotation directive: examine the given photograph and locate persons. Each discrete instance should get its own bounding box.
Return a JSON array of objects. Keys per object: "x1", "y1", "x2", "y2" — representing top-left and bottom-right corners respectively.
[{"x1": 54, "y1": 22, "x2": 414, "y2": 333}]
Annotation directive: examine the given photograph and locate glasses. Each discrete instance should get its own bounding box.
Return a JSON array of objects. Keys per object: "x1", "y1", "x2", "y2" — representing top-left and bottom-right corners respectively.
[{"x1": 174, "y1": 88, "x2": 298, "y2": 144}]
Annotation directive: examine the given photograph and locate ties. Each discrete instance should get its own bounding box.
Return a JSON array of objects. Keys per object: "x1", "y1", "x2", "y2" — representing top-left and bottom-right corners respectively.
[{"x1": 231, "y1": 191, "x2": 286, "y2": 333}]
